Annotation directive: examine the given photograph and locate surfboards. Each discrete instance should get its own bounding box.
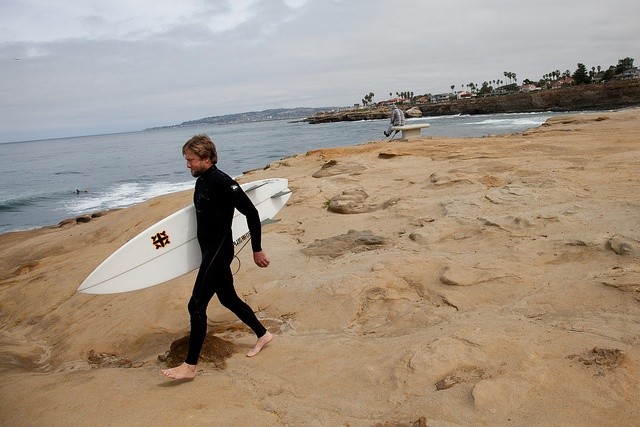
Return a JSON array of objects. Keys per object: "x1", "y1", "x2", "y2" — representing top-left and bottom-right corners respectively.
[{"x1": 78, "y1": 178, "x2": 296, "y2": 294}]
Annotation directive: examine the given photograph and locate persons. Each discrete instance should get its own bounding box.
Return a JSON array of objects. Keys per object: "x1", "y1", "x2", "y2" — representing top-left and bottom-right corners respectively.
[
  {"x1": 384, "y1": 104, "x2": 406, "y2": 137},
  {"x1": 160, "y1": 134, "x2": 273, "y2": 381}
]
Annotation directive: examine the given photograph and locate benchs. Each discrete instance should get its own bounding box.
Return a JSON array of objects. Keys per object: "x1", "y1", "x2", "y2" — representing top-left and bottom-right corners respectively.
[{"x1": 391, "y1": 123, "x2": 431, "y2": 139}]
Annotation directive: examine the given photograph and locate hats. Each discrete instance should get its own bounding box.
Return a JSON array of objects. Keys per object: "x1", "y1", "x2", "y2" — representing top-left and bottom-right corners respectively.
[{"x1": 391, "y1": 105, "x2": 397, "y2": 109}]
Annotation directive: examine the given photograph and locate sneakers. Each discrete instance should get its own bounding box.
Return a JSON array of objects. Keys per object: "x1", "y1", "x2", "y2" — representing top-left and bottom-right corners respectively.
[{"x1": 384, "y1": 131, "x2": 389, "y2": 137}]
